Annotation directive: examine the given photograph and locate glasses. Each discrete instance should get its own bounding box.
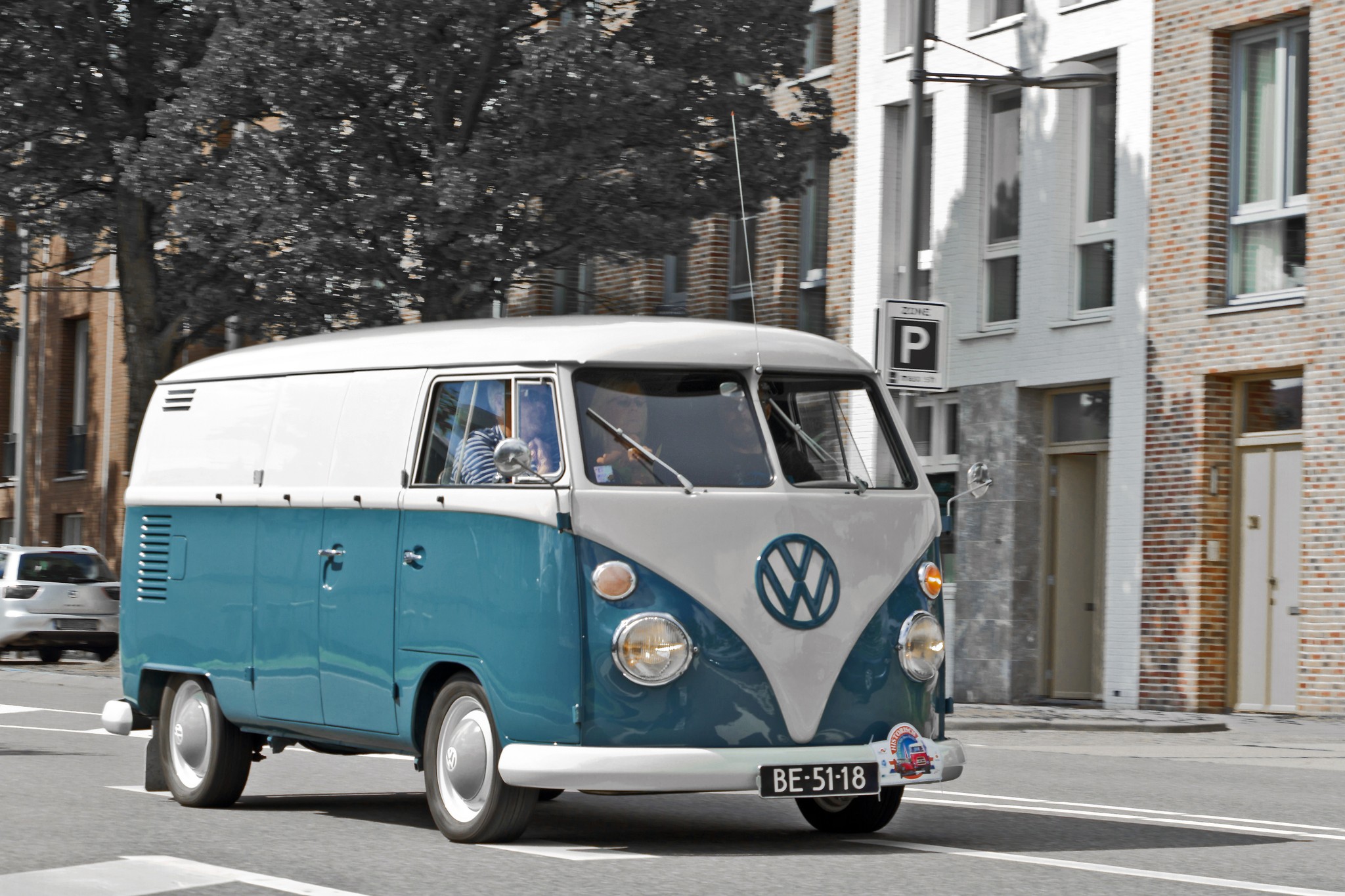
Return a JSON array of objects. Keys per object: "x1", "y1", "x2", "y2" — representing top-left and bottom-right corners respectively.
[
  {"x1": 760, "y1": 398, "x2": 769, "y2": 407},
  {"x1": 605, "y1": 393, "x2": 648, "y2": 409},
  {"x1": 500, "y1": 387, "x2": 553, "y2": 406}
]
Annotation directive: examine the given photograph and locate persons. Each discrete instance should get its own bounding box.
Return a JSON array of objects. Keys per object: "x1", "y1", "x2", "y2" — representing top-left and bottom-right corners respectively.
[
  {"x1": 583, "y1": 376, "x2": 672, "y2": 487},
  {"x1": 452, "y1": 378, "x2": 557, "y2": 487},
  {"x1": 684, "y1": 383, "x2": 825, "y2": 489}
]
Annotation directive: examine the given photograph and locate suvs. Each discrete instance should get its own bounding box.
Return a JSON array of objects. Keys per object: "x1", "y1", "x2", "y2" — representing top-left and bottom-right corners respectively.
[{"x1": 0, "y1": 543, "x2": 122, "y2": 664}]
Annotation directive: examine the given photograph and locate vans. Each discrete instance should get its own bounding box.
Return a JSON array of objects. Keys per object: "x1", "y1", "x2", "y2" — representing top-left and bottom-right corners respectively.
[{"x1": 96, "y1": 314, "x2": 997, "y2": 843}]
